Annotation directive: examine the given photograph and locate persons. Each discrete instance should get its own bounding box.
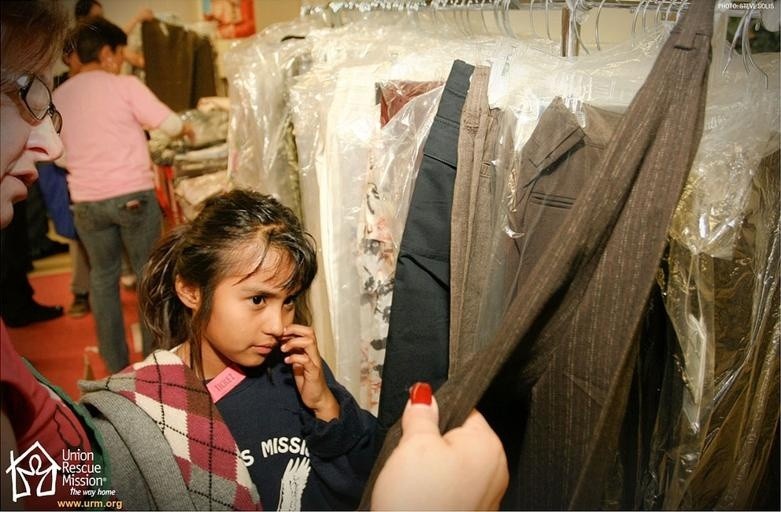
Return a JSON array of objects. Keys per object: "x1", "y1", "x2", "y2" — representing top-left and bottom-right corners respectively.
[
  {"x1": 49, "y1": 16, "x2": 197, "y2": 374},
  {"x1": 0, "y1": 199, "x2": 65, "y2": 329},
  {"x1": 78, "y1": 188, "x2": 388, "y2": 511},
  {"x1": 26, "y1": 177, "x2": 70, "y2": 261},
  {"x1": 62, "y1": 26, "x2": 91, "y2": 317},
  {"x1": 1, "y1": 0, "x2": 513, "y2": 510},
  {"x1": 74, "y1": 1, "x2": 154, "y2": 68}
]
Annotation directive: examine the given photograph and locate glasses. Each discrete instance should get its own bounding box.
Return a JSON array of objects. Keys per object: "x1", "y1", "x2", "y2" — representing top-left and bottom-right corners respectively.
[{"x1": 0, "y1": 69, "x2": 63, "y2": 137}]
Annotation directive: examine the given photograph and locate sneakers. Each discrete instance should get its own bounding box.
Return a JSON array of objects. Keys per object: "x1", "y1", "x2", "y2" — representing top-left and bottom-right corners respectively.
[{"x1": 66, "y1": 290, "x2": 92, "y2": 320}]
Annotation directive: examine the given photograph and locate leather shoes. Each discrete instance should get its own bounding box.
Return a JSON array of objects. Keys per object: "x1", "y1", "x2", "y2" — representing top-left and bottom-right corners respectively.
[{"x1": 0, "y1": 302, "x2": 63, "y2": 329}]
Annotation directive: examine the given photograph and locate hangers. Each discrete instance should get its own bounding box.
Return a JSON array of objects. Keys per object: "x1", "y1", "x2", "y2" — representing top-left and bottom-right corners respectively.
[{"x1": 338, "y1": 0, "x2": 777, "y2": 96}]
